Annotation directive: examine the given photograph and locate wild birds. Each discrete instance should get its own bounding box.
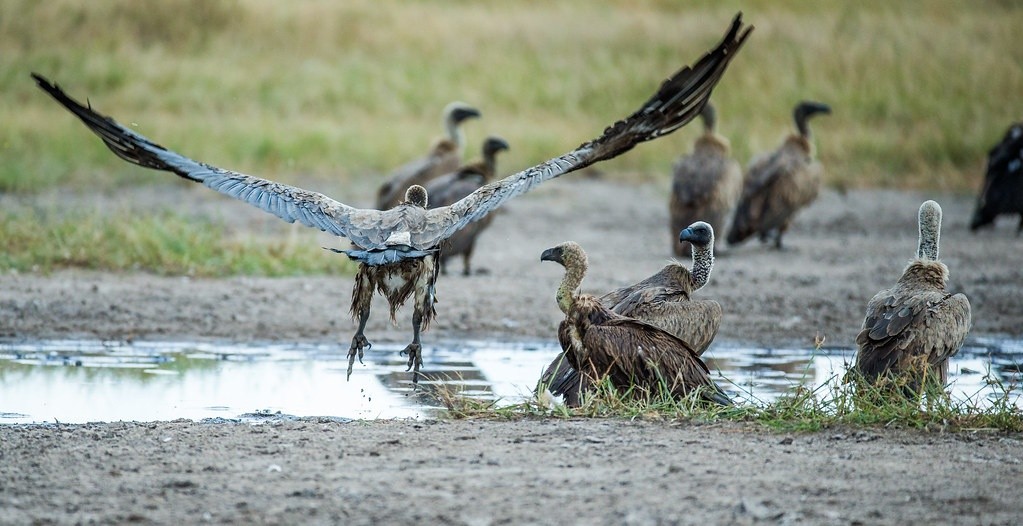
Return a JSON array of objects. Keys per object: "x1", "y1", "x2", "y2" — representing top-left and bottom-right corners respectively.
[
  {"x1": 728, "y1": 99, "x2": 832, "y2": 250},
  {"x1": 29, "y1": 11, "x2": 755, "y2": 407},
  {"x1": 969, "y1": 119, "x2": 1023, "y2": 236},
  {"x1": 842, "y1": 200, "x2": 971, "y2": 414}
]
